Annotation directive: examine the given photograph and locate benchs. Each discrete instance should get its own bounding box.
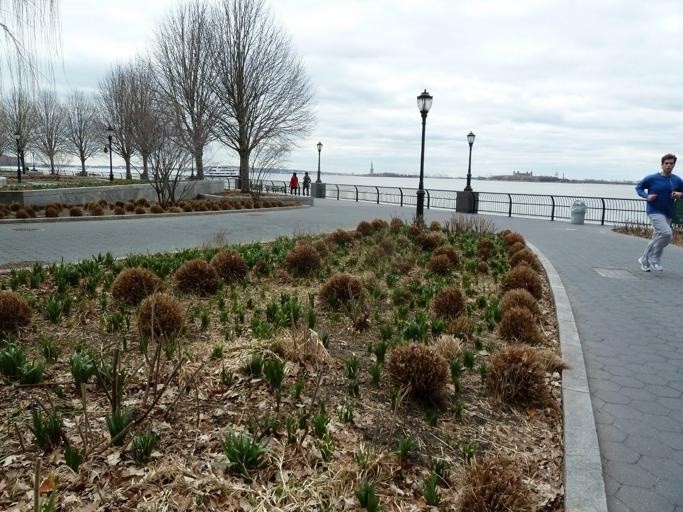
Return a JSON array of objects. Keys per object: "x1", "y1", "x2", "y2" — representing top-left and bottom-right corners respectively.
[
  {"x1": 252, "y1": 184, "x2": 263, "y2": 193},
  {"x1": 265, "y1": 185, "x2": 285, "y2": 194}
]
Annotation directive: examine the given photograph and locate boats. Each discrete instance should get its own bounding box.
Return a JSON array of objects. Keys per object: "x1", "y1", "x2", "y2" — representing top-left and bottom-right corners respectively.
[{"x1": 204, "y1": 165, "x2": 240, "y2": 177}]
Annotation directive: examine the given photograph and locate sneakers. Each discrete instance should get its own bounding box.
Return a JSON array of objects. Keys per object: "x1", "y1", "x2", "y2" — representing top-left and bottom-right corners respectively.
[{"x1": 639, "y1": 257, "x2": 663, "y2": 272}]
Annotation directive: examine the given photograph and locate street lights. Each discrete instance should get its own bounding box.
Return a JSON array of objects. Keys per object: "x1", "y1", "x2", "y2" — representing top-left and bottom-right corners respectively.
[
  {"x1": 463, "y1": 131, "x2": 476, "y2": 191},
  {"x1": 416, "y1": 88, "x2": 433, "y2": 228},
  {"x1": 316, "y1": 142, "x2": 323, "y2": 182},
  {"x1": 13, "y1": 130, "x2": 21, "y2": 182},
  {"x1": 104, "y1": 125, "x2": 115, "y2": 182}
]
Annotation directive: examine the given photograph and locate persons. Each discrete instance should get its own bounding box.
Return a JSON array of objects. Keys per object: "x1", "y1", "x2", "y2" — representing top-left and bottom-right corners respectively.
[
  {"x1": 635, "y1": 153, "x2": 683, "y2": 272},
  {"x1": 289, "y1": 173, "x2": 298, "y2": 195},
  {"x1": 302, "y1": 172, "x2": 311, "y2": 196}
]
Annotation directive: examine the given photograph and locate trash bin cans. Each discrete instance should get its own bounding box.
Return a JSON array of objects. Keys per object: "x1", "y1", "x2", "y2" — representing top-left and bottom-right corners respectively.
[{"x1": 571, "y1": 200, "x2": 585, "y2": 224}]
